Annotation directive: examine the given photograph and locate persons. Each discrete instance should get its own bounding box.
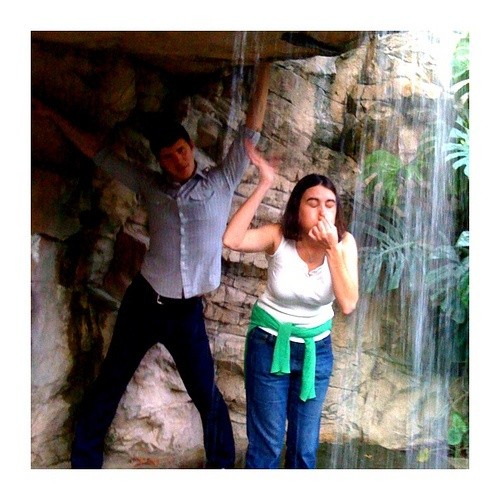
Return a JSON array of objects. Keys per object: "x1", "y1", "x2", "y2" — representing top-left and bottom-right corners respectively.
[
  {"x1": 221, "y1": 139, "x2": 358, "y2": 469},
  {"x1": 31, "y1": 57, "x2": 237, "y2": 470}
]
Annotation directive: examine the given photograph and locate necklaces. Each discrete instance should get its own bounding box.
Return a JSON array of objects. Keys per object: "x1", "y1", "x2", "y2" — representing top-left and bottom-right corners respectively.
[{"x1": 301, "y1": 238, "x2": 326, "y2": 263}]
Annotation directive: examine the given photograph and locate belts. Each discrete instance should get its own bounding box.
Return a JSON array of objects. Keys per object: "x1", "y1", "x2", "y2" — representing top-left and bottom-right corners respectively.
[{"x1": 138, "y1": 274, "x2": 203, "y2": 305}]
